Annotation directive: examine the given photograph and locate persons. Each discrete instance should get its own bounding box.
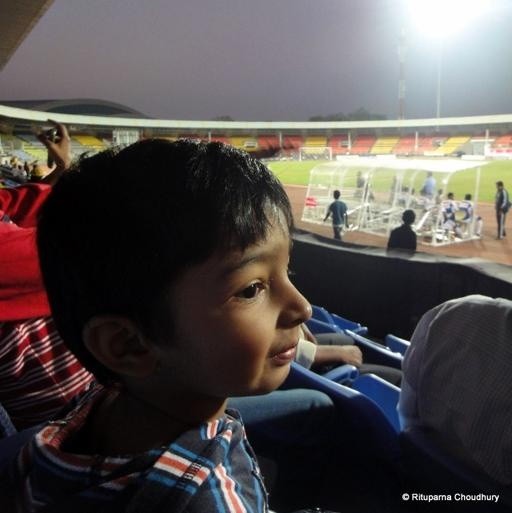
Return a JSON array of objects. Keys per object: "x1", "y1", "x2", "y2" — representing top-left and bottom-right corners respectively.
[
  {"x1": 322, "y1": 189, "x2": 350, "y2": 241},
  {"x1": 386, "y1": 208, "x2": 417, "y2": 251},
  {"x1": 291, "y1": 317, "x2": 403, "y2": 387},
  {"x1": 492, "y1": 179, "x2": 509, "y2": 239},
  {"x1": 390, "y1": 169, "x2": 475, "y2": 239},
  {"x1": 379, "y1": 292, "x2": 510, "y2": 510},
  {"x1": 1, "y1": 153, "x2": 45, "y2": 182},
  {"x1": 355, "y1": 170, "x2": 365, "y2": 188}
]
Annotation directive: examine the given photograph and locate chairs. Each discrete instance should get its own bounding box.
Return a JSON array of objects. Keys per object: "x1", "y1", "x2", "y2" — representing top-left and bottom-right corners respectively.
[{"x1": 274, "y1": 299, "x2": 494, "y2": 512}]
